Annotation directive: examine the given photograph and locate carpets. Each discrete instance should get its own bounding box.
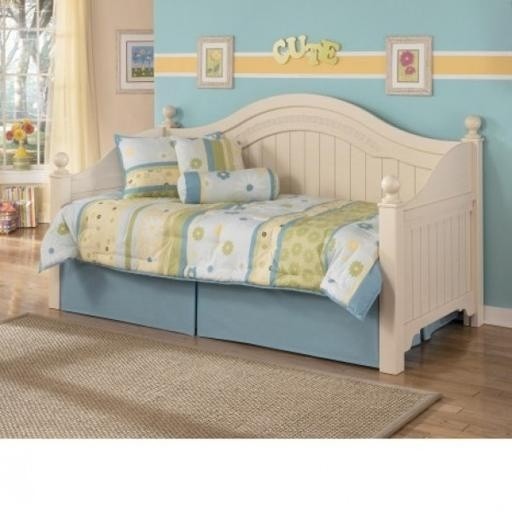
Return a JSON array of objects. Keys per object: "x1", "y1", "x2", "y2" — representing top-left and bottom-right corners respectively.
[{"x1": 0, "y1": 312, "x2": 443, "y2": 438}]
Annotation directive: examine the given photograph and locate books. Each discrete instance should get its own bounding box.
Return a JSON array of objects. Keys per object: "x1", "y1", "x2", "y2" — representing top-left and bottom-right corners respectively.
[{"x1": 0, "y1": 185, "x2": 39, "y2": 235}]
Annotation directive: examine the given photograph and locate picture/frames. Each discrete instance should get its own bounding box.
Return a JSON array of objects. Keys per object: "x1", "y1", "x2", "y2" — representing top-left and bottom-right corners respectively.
[
  {"x1": 115, "y1": 28, "x2": 154, "y2": 93},
  {"x1": 197, "y1": 35, "x2": 234, "y2": 89},
  {"x1": 386, "y1": 35, "x2": 433, "y2": 96}
]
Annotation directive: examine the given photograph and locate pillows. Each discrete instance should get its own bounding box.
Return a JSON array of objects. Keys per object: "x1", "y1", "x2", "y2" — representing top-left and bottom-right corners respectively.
[
  {"x1": 170, "y1": 135, "x2": 245, "y2": 171},
  {"x1": 113, "y1": 134, "x2": 180, "y2": 198},
  {"x1": 176, "y1": 166, "x2": 279, "y2": 205}
]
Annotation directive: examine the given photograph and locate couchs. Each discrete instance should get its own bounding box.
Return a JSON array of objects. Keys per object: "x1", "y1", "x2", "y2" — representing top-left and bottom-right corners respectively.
[{"x1": 37, "y1": 92, "x2": 485, "y2": 377}]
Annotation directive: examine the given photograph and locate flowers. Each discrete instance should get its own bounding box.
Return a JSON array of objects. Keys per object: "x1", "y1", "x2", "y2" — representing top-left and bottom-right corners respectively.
[{"x1": 4, "y1": 118, "x2": 35, "y2": 158}]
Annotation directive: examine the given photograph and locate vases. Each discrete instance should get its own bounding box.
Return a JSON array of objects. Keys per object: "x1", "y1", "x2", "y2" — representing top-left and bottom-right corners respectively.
[{"x1": 11, "y1": 158, "x2": 34, "y2": 171}]
